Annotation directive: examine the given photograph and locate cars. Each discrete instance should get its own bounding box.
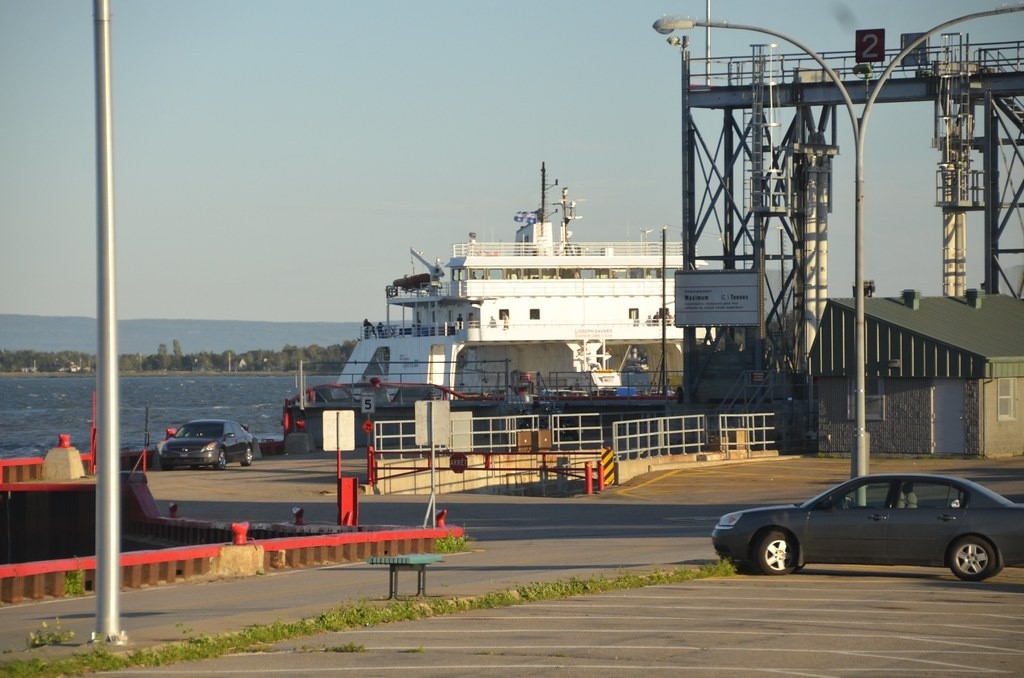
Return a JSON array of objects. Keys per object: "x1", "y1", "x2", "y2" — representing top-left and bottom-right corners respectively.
[
  {"x1": 710, "y1": 472, "x2": 1023, "y2": 582},
  {"x1": 157, "y1": 418, "x2": 255, "y2": 471}
]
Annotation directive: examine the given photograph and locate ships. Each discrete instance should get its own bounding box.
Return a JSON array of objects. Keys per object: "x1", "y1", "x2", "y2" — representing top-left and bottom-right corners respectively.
[{"x1": 280, "y1": 161, "x2": 719, "y2": 448}]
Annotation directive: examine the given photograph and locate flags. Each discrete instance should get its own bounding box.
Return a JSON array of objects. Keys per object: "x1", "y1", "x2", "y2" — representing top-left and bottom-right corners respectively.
[{"x1": 513, "y1": 209, "x2": 542, "y2": 225}]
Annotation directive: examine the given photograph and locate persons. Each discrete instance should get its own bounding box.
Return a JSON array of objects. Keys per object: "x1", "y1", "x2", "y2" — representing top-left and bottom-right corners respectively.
[
  {"x1": 674, "y1": 386, "x2": 684, "y2": 403},
  {"x1": 363, "y1": 318, "x2": 378, "y2": 339},
  {"x1": 376, "y1": 321, "x2": 385, "y2": 338}
]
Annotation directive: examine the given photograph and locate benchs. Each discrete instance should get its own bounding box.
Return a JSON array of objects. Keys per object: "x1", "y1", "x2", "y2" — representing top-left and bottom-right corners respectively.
[{"x1": 366, "y1": 554, "x2": 445, "y2": 601}]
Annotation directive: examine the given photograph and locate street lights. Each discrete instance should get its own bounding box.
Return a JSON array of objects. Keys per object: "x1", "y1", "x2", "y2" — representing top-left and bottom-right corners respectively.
[{"x1": 654, "y1": 5, "x2": 1024, "y2": 507}]
